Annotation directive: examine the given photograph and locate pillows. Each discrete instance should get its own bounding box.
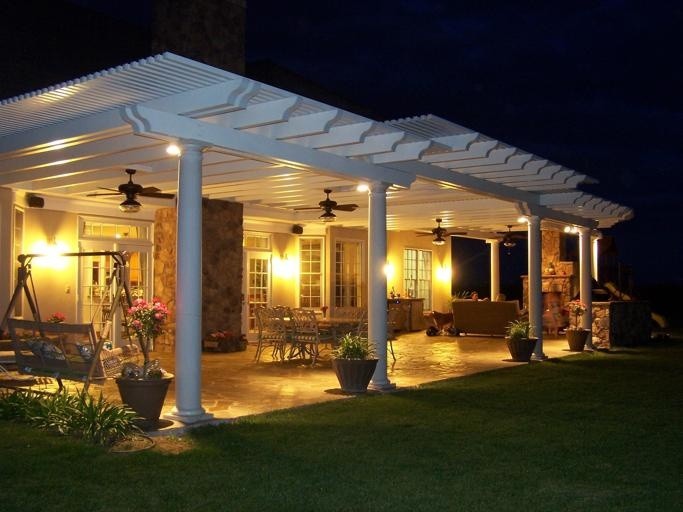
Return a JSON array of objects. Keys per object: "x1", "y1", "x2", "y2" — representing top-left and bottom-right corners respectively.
[{"x1": 24, "y1": 339, "x2": 120, "y2": 369}]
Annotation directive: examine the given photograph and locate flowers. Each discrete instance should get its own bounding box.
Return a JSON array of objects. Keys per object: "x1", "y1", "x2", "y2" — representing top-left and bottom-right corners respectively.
[
  {"x1": 122, "y1": 299, "x2": 173, "y2": 379},
  {"x1": 566, "y1": 300, "x2": 588, "y2": 329}
]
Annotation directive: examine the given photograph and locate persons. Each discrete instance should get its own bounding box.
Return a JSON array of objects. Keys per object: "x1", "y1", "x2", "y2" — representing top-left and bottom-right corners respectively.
[{"x1": 469, "y1": 290, "x2": 478, "y2": 300}]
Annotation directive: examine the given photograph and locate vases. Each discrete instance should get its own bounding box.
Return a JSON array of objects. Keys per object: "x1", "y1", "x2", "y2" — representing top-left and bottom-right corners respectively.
[
  {"x1": 114, "y1": 374, "x2": 175, "y2": 423},
  {"x1": 564, "y1": 328, "x2": 590, "y2": 352}
]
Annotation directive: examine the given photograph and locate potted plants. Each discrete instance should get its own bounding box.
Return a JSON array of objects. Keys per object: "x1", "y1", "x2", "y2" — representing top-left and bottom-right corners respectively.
[
  {"x1": 332, "y1": 332, "x2": 380, "y2": 392},
  {"x1": 505, "y1": 320, "x2": 539, "y2": 361}
]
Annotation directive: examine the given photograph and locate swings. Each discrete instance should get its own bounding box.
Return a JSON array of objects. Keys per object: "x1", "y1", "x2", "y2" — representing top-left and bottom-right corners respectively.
[{"x1": 6, "y1": 270, "x2": 144, "y2": 385}]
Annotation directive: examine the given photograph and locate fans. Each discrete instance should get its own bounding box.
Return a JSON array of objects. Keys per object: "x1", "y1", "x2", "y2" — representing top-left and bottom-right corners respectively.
[
  {"x1": 293, "y1": 189, "x2": 359, "y2": 213},
  {"x1": 86, "y1": 171, "x2": 175, "y2": 199},
  {"x1": 488, "y1": 225, "x2": 528, "y2": 240},
  {"x1": 414, "y1": 219, "x2": 468, "y2": 238}
]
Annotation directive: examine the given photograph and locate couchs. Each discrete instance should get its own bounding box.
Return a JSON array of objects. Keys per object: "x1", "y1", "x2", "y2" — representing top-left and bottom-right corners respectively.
[{"x1": 452, "y1": 299, "x2": 529, "y2": 337}]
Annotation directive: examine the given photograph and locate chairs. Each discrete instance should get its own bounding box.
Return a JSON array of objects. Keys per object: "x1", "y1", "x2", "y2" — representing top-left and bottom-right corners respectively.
[{"x1": 253, "y1": 305, "x2": 400, "y2": 367}]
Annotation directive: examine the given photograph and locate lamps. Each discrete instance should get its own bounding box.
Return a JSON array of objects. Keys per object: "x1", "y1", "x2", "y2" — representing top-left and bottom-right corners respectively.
[
  {"x1": 319, "y1": 213, "x2": 337, "y2": 222},
  {"x1": 432, "y1": 239, "x2": 446, "y2": 245},
  {"x1": 118, "y1": 200, "x2": 142, "y2": 213},
  {"x1": 504, "y1": 240, "x2": 516, "y2": 247}
]
